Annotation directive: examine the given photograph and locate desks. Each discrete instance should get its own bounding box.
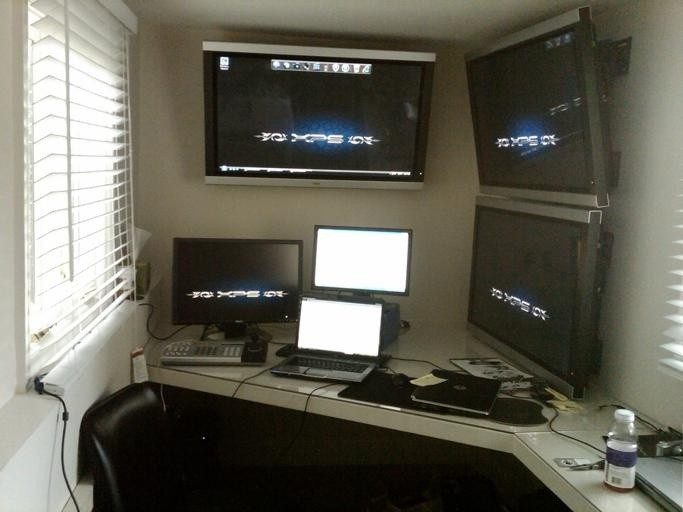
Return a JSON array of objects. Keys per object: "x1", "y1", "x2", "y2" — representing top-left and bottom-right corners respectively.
[{"x1": 142, "y1": 320, "x2": 670, "y2": 512}]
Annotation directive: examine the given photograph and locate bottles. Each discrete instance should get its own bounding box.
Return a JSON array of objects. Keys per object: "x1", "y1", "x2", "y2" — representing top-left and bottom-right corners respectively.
[{"x1": 604, "y1": 409, "x2": 638, "y2": 493}]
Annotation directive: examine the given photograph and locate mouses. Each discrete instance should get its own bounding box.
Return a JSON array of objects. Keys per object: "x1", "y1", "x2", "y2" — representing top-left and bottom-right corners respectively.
[{"x1": 391, "y1": 371, "x2": 410, "y2": 388}]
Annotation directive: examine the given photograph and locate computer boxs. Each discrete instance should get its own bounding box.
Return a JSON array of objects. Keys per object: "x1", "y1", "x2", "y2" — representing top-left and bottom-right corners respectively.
[{"x1": 380, "y1": 302, "x2": 402, "y2": 352}]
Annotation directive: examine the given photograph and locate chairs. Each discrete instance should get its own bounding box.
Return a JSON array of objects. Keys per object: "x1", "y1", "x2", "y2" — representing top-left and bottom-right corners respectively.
[{"x1": 83, "y1": 382, "x2": 161, "y2": 512}]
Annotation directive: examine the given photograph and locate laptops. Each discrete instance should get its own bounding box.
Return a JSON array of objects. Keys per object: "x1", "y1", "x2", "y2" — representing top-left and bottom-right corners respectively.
[
  {"x1": 410, "y1": 368, "x2": 503, "y2": 416},
  {"x1": 268, "y1": 297, "x2": 386, "y2": 384}
]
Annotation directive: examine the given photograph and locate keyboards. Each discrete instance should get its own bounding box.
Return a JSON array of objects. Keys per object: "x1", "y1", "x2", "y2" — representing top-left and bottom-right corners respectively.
[
  {"x1": 276, "y1": 344, "x2": 392, "y2": 367},
  {"x1": 162, "y1": 339, "x2": 246, "y2": 362}
]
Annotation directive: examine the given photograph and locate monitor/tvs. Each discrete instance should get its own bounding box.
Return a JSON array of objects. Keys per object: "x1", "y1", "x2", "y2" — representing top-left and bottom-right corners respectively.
[
  {"x1": 171, "y1": 236, "x2": 299, "y2": 344},
  {"x1": 465, "y1": 6, "x2": 632, "y2": 210},
  {"x1": 199, "y1": 40, "x2": 435, "y2": 190},
  {"x1": 311, "y1": 225, "x2": 412, "y2": 294},
  {"x1": 465, "y1": 192, "x2": 611, "y2": 401}
]
